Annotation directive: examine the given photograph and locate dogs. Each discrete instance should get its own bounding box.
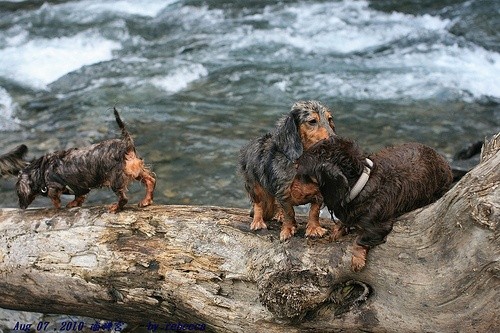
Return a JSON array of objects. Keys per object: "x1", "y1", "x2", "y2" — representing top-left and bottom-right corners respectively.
[
  {"x1": 238, "y1": 98, "x2": 337, "y2": 244},
  {"x1": 295, "y1": 133, "x2": 453, "y2": 274},
  {"x1": 15, "y1": 106, "x2": 158, "y2": 214}
]
